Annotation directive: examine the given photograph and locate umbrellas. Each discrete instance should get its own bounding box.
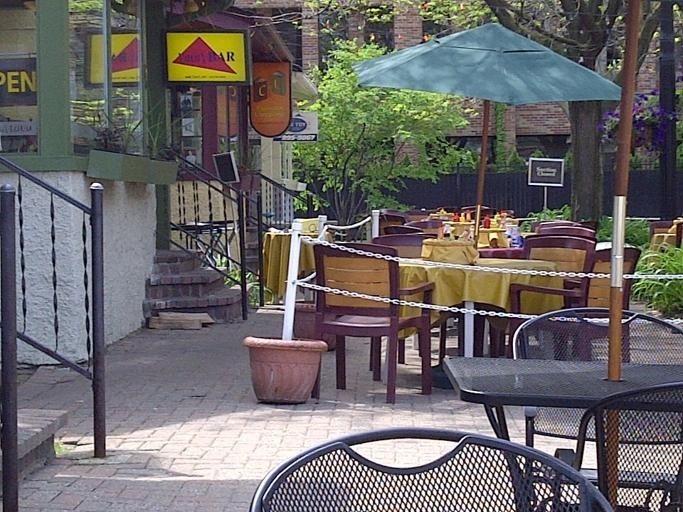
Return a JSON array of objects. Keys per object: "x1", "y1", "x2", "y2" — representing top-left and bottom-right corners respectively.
[{"x1": 350, "y1": 17, "x2": 621, "y2": 251}]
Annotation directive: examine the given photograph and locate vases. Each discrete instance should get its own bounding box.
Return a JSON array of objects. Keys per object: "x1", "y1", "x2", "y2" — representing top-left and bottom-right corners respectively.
[{"x1": 242, "y1": 335, "x2": 329, "y2": 405}]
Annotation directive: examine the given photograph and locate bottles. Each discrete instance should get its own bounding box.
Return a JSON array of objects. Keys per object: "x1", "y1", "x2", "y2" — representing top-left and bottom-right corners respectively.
[{"x1": 428, "y1": 207, "x2": 524, "y2": 249}]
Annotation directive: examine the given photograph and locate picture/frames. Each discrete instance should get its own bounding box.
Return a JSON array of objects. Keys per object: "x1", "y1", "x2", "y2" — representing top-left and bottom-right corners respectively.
[
  {"x1": 85, "y1": 31, "x2": 140, "y2": 89},
  {"x1": 528, "y1": 157, "x2": 565, "y2": 187},
  {"x1": 161, "y1": 29, "x2": 251, "y2": 86}
]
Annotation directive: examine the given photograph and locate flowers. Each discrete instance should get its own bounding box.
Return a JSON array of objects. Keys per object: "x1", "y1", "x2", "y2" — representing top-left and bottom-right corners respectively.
[{"x1": 595, "y1": 87, "x2": 683, "y2": 170}]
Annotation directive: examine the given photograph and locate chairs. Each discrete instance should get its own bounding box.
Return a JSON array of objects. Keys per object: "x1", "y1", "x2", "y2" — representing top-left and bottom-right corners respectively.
[{"x1": 249, "y1": 422, "x2": 611, "y2": 511}]
